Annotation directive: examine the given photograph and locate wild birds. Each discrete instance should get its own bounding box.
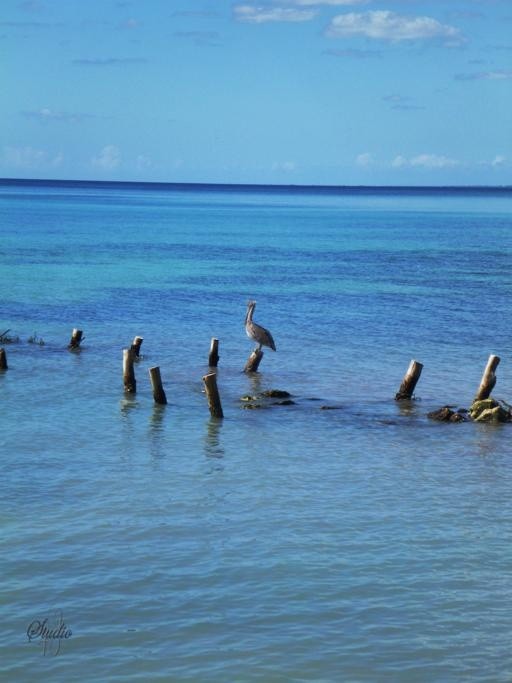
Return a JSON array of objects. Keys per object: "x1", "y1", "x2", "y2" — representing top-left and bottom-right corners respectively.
[{"x1": 243, "y1": 299, "x2": 277, "y2": 352}]
